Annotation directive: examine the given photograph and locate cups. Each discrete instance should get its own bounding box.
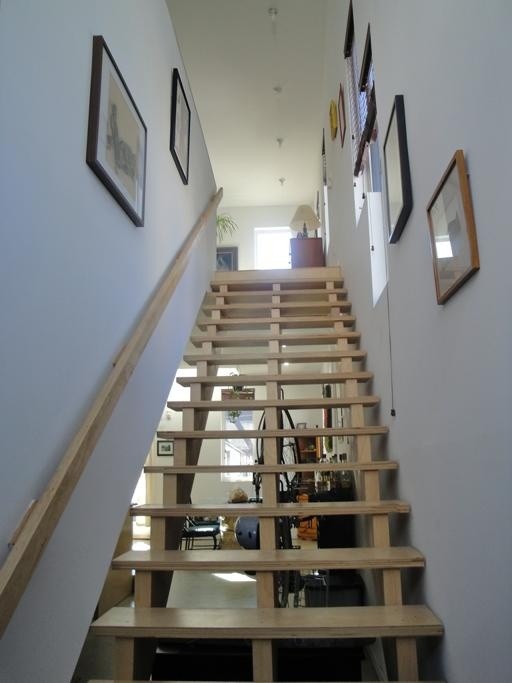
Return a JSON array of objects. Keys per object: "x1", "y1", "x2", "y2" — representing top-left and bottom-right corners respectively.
[{"x1": 297, "y1": 422, "x2": 306, "y2": 428}]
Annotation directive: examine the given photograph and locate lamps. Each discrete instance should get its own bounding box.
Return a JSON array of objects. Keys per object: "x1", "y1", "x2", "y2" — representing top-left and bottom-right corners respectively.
[{"x1": 290, "y1": 204, "x2": 321, "y2": 236}]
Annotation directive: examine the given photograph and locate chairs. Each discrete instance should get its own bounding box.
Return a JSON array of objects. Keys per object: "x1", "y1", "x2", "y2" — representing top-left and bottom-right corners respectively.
[{"x1": 179, "y1": 515, "x2": 221, "y2": 550}]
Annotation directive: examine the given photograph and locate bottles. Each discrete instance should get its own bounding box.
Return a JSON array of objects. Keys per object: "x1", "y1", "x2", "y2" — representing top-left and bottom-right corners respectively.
[{"x1": 315, "y1": 454, "x2": 354, "y2": 491}]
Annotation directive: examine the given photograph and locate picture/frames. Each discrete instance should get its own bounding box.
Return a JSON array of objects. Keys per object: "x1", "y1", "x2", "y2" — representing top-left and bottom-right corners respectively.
[
  {"x1": 330, "y1": 82, "x2": 480, "y2": 305},
  {"x1": 86, "y1": 34, "x2": 147, "y2": 226},
  {"x1": 216, "y1": 247, "x2": 238, "y2": 271},
  {"x1": 157, "y1": 440, "x2": 174, "y2": 456},
  {"x1": 170, "y1": 67, "x2": 191, "y2": 184}
]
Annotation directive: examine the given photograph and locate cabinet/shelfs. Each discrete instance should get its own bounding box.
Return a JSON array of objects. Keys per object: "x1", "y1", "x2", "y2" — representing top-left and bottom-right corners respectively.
[
  {"x1": 288, "y1": 237, "x2": 325, "y2": 268},
  {"x1": 315, "y1": 488, "x2": 357, "y2": 549}
]
